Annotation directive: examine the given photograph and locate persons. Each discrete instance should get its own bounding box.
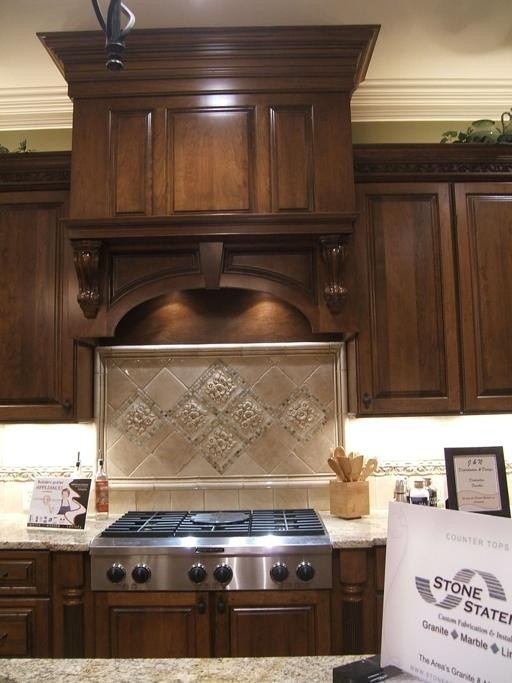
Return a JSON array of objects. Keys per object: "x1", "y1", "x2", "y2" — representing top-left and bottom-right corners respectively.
[{"x1": 49, "y1": 486, "x2": 72, "y2": 515}]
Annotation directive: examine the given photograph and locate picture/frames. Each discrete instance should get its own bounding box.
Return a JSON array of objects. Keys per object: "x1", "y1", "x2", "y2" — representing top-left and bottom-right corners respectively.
[{"x1": 443, "y1": 445, "x2": 512, "y2": 519}]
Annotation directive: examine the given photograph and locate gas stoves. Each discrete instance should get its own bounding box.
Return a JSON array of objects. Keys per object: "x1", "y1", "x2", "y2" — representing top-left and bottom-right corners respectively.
[{"x1": 90, "y1": 509, "x2": 334, "y2": 589}]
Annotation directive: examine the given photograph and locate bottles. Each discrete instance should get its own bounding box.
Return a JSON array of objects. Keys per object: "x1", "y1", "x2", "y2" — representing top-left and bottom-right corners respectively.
[
  {"x1": 93, "y1": 459, "x2": 109, "y2": 521},
  {"x1": 409, "y1": 479, "x2": 430, "y2": 507},
  {"x1": 422, "y1": 478, "x2": 438, "y2": 508}
]
